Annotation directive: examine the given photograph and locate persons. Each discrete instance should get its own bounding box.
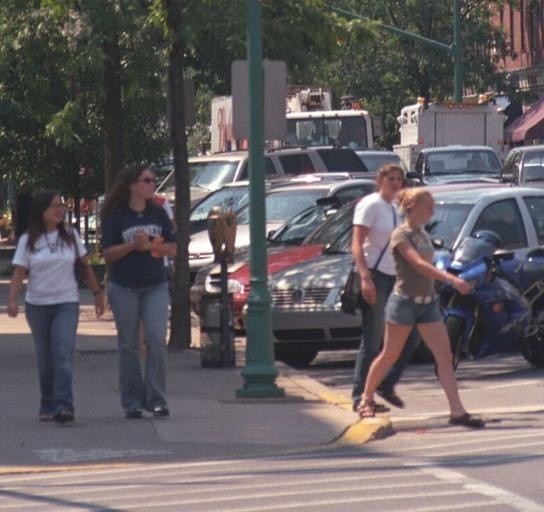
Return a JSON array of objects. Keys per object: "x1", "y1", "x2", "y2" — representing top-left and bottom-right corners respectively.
[
  {"x1": 357, "y1": 188, "x2": 485, "y2": 428},
  {"x1": 7, "y1": 191, "x2": 104, "y2": 425},
  {"x1": 100, "y1": 164, "x2": 178, "y2": 419},
  {"x1": 140, "y1": 197, "x2": 176, "y2": 362},
  {"x1": 351, "y1": 164, "x2": 423, "y2": 413}
]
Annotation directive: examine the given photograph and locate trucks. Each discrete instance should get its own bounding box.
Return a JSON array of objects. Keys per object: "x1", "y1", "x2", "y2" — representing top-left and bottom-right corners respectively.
[
  {"x1": 393, "y1": 97, "x2": 505, "y2": 160},
  {"x1": 211, "y1": 85, "x2": 374, "y2": 154}
]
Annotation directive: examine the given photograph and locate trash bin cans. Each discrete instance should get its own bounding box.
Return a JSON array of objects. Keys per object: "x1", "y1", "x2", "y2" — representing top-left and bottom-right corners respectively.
[{"x1": 199, "y1": 292, "x2": 236, "y2": 368}]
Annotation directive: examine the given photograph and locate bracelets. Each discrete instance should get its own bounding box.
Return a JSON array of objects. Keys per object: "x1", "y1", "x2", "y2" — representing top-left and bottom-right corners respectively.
[
  {"x1": 450, "y1": 277, "x2": 455, "y2": 286},
  {"x1": 93, "y1": 291, "x2": 101, "y2": 296}
]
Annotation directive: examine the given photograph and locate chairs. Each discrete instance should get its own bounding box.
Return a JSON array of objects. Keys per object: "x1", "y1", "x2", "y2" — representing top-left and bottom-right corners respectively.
[
  {"x1": 484, "y1": 218, "x2": 518, "y2": 244},
  {"x1": 466, "y1": 159, "x2": 488, "y2": 169},
  {"x1": 429, "y1": 160, "x2": 445, "y2": 170},
  {"x1": 526, "y1": 167, "x2": 544, "y2": 178}
]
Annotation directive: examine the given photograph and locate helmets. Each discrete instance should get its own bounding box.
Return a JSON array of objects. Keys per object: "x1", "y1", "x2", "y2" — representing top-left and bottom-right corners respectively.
[{"x1": 473, "y1": 230, "x2": 502, "y2": 248}]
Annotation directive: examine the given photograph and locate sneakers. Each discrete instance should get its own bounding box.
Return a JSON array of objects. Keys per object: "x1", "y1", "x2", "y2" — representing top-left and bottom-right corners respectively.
[
  {"x1": 375, "y1": 387, "x2": 402, "y2": 407},
  {"x1": 353, "y1": 400, "x2": 386, "y2": 412},
  {"x1": 125, "y1": 404, "x2": 143, "y2": 418},
  {"x1": 151, "y1": 404, "x2": 169, "y2": 417}
]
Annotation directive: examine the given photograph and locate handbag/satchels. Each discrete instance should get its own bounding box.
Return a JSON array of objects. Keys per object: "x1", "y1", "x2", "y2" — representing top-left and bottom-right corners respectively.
[{"x1": 341, "y1": 271, "x2": 362, "y2": 308}]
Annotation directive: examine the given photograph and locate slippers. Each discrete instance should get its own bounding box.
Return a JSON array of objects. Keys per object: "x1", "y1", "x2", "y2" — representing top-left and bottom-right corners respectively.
[
  {"x1": 449, "y1": 410, "x2": 482, "y2": 427},
  {"x1": 358, "y1": 398, "x2": 375, "y2": 418},
  {"x1": 40, "y1": 408, "x2": 74, "y2": 423}
]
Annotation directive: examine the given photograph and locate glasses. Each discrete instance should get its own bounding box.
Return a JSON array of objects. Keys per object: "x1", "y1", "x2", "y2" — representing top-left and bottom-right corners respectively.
[{"x1": 135, "y1": 177, "x2": 154, "y2": 183}]
